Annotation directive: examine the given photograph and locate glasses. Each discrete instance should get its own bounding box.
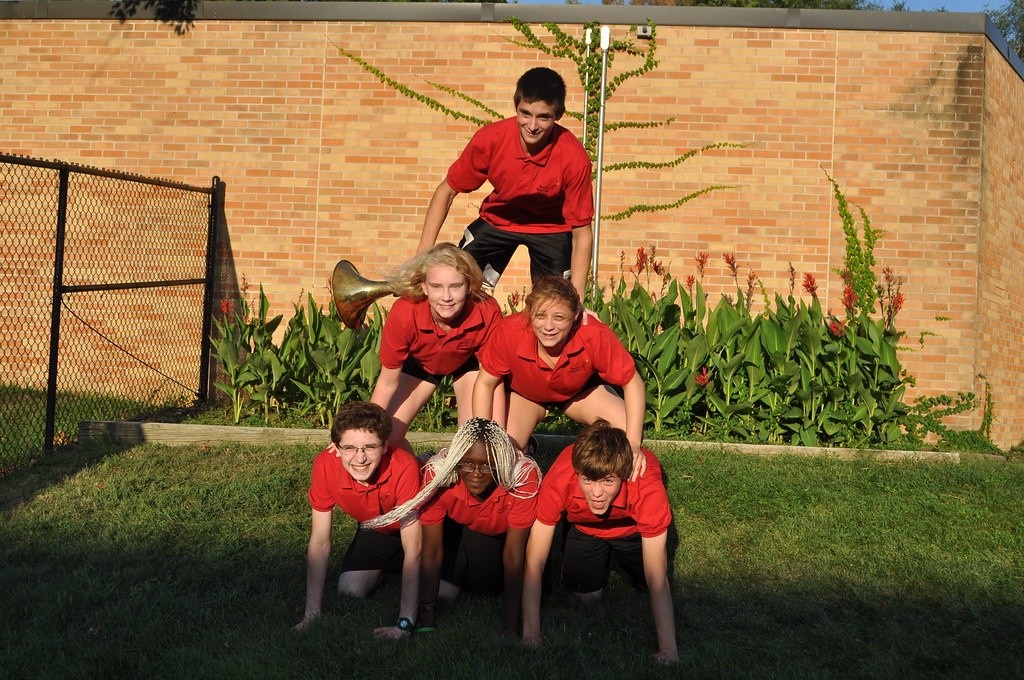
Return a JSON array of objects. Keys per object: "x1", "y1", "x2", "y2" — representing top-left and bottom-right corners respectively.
[
  {"x1": 339, "y1": 444, "x2": 382, "y2": 454},
  {"x1": 457, "y1": 463, "x2": 496, "y2": 474}
]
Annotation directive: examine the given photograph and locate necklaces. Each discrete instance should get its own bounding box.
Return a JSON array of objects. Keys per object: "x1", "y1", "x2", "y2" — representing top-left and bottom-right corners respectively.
[
  {"x1": 539, "y1": 346, "x2": 555, "y2": 366},
  {"x1": 433, "y1": 317, "x2": 440, "y2": 327}
]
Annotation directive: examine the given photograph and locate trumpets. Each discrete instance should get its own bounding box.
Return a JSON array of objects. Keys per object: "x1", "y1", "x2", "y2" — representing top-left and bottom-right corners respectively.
[{"x1": 332, "y1": 259, "x2": 402, "y2": 331}]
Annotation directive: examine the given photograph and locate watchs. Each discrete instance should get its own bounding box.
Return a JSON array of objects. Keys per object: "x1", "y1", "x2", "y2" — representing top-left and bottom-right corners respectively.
[{"x1": 396, "y1": 617, "x2": 416, "y2": 635}]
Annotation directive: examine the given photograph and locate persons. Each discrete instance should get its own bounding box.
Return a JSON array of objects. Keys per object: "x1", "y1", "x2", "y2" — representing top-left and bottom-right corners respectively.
[
  {"x1": 324, "y1": 242, "x2": 512, "y2": 474},
  {"x1": 359, "y1": 417, "x2": 543, "y2": 641},
  {"x1": 288, "y1": 400, "x2": 459, "y2": 645},
  {"x1": 472, "y1": 275, "x2": 649, "y2": 482},
  {"x1": 417, "y1": 66, "x2": 604, "y2": 326},
  {"x1": 516, "y1": 416, "x2": 682, "y2": 668}
]
